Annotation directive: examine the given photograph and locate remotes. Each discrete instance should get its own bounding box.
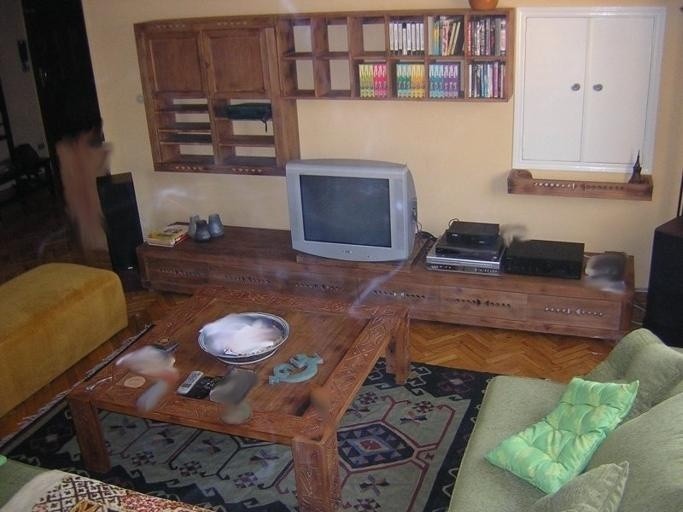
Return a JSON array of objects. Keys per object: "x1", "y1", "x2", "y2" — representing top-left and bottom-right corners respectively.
[
  {"x1": 195, "y1": 376, "x2": 225, "y2": 399},
  {"x1": 176, "y1": 370, "x2": 204, "y2": 395},
  {"x1": 187, "y1": 377, "x2": 211, "y2": 398}
]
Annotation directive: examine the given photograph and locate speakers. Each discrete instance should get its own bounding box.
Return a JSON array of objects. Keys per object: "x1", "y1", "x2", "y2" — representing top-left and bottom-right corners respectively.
[
  {"x1": 95, "y1": 172, "x2": 144, "y2": 293},
  {"x1": 510, "y1": 239, "x2": 585, "y2": 280},
  {"x1": 642, "y1": 216, "x2": 683, "y2": 348}
]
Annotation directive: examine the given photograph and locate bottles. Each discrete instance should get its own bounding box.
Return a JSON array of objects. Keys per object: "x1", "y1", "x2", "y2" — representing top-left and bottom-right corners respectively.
[
  {"x1": 208, "y1": 214, "x2": 224, "y2": 238},
  {"x1": 186, "y1": 215, "x2": 199, "y2": 239},
  {"x1": 193, "y1": 219, "x2": 211, "y2": 241}
]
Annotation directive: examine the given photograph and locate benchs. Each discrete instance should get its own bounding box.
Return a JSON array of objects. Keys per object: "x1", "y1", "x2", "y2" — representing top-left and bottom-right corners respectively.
[{"x1": 0, "y1": 262, "x2": 128, "y2": 414}]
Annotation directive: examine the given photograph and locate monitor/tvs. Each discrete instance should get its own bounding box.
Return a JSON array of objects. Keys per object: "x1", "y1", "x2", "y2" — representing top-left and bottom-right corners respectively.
[{"x1": 285, "y1": 158, "x2": 418, "y2": 263}]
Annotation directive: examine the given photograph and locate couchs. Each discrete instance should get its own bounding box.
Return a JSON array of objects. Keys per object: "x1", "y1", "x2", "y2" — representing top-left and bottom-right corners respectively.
[{"x1": 444, "y1": 325, "x2": 683, "y2": 512}]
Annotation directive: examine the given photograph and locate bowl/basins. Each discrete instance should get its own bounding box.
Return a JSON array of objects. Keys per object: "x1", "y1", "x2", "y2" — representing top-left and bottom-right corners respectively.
[{"x1": 196, "y1": 311, "x2": 290, "y2": 365}]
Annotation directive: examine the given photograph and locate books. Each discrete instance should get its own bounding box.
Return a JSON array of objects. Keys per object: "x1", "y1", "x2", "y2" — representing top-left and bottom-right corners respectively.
[
  {"x1": 357, "y1": 62, "x2": 507, "y2": 100},
  {"x1": 147, "y1": 223, "x2": 189, "y2": 248},
  {"x1": 388, "y1": 16, "x2": 506, "y2": 58}
]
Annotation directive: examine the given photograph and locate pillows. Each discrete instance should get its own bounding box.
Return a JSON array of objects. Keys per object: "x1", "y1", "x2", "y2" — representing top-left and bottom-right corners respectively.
[{"x1": 480, "y1": 377, "x2": 640, "y2": 511}]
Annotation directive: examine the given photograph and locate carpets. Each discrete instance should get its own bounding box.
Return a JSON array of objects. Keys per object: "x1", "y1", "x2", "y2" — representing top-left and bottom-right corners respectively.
[{"x1": 1, "y1": 359, "x2": 504, "y2": 511}]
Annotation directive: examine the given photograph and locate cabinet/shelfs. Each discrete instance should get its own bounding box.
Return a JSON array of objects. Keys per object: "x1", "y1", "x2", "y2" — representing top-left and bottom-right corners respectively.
[
  {"x1": 141, "y1": 13, "x2": 299, "y2": 175},
  {"x1": 512, "y1": 8, "x2": 666, "y2": 174},
  {"x1": 278, "y1": 8, "x2": 512, "y2": 101}
]
